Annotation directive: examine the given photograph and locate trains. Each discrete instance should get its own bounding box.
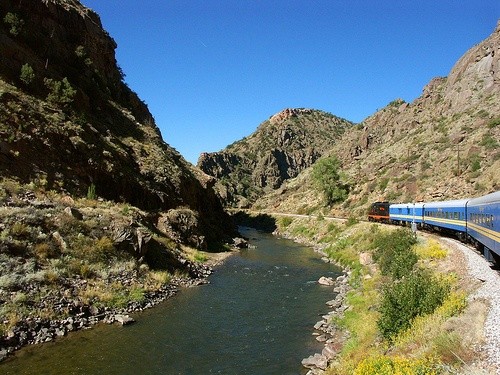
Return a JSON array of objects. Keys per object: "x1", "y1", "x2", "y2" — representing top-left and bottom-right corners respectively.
[{"x1": 368, "y1": 191, "x2": 500, "y2": 269}]
